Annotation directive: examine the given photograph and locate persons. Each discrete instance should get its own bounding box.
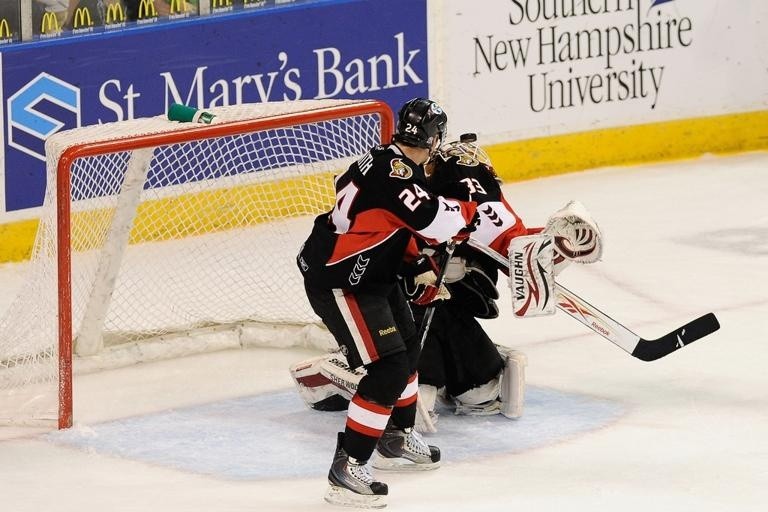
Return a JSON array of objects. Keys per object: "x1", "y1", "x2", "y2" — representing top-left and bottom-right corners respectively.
[{"x1": 2, "y1": 0, "x2": 205, "y2": 39}]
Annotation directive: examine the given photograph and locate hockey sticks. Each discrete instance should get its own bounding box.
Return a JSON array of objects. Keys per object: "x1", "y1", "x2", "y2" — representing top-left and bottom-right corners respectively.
[{"x1": 464, "y1": 235, "x2": 721, "y2": 361}]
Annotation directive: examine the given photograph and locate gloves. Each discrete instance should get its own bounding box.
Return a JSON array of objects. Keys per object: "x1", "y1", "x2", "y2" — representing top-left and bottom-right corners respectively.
[{"x1": 398, "y1": 252, "x2": 453, "y2": 308}]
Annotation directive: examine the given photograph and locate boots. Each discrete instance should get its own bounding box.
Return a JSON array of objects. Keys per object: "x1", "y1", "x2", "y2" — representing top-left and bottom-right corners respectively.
[
  {"x1": 326, "y1": 430, "x2": 388, "y2": 495},
  {"x1": 377, "y1": 420, "x2": 440, "y2": 463}
]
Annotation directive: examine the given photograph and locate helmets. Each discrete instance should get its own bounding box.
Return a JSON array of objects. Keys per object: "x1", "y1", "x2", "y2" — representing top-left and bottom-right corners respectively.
[{"x1": 398, "y1": 97, "x2": 448, "y2": 149}]
